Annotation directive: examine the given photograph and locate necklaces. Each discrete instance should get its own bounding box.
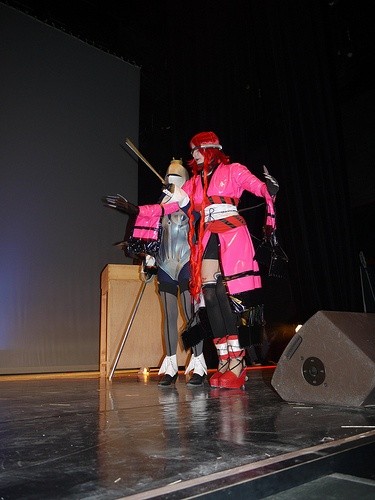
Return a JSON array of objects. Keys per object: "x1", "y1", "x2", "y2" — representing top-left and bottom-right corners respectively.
[{"x1": 202, "y1": 167, "x2": 214, "y2": 177}]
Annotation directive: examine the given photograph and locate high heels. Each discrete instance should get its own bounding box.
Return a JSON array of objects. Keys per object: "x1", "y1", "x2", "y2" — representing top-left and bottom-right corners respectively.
[
  {"x1": 218, "y1": 366, "x2": 247, "y2": 388},
  {"x1": 210, "y1": 370, "x2": 226, "y2": 387}
]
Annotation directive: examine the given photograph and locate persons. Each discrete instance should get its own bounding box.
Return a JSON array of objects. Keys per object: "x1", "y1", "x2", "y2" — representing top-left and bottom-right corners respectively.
[
  {"x1": 102, "y1": 131, "x2": 277, "y2": 389},
  {"x1": 154, "y1": 160, "x2": 202, "y2": 387}
]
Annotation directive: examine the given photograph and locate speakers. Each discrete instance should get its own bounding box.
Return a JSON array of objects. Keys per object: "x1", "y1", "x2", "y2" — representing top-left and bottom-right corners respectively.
[{"x1": 271, "y1": 311, "x2": 375, "y2": 408}]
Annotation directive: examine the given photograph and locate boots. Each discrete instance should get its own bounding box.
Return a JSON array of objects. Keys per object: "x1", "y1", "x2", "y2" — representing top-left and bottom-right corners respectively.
[
  {"x1": 184, "y1": 353, "x2": 207, "y2": 384},
  {"x1": 158, "y1": 354, "x2": 178, "y2": 385}
]
